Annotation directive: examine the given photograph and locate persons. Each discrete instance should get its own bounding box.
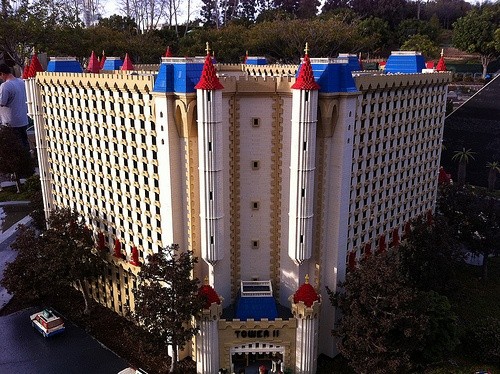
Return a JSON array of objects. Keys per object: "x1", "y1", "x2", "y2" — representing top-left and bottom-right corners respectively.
[
  {"x1": 218, "y1": 363, "x2": 268, "y2": 374},
  {"x1": 5, "y1": 59, "x2": 21, "y2": 78},
  {"x1": 0, "y1": 62, "x2": 36, "y2": 178}
]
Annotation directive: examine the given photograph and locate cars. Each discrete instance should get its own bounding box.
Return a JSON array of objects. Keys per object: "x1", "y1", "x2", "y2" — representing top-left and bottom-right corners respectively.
[{"x1": 29, "y1": 308, "x2": 66, "y2": 338}]
